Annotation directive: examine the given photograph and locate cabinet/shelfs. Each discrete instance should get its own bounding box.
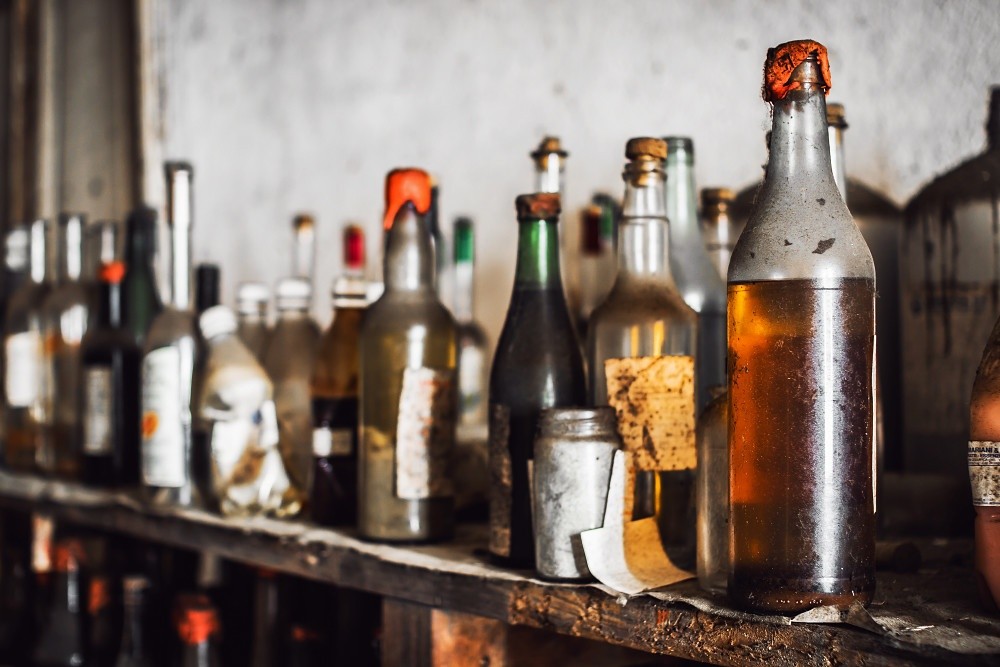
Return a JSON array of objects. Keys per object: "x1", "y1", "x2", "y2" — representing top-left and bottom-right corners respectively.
[{"x1": 0, "y1": 462, "x2": 1000, "y2": 667}]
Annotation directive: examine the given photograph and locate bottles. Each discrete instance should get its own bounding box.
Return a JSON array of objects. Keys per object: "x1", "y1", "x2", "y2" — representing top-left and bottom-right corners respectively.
[{"x1": 0, "y1": 41, "x2": 1000, "y2": 666}]
